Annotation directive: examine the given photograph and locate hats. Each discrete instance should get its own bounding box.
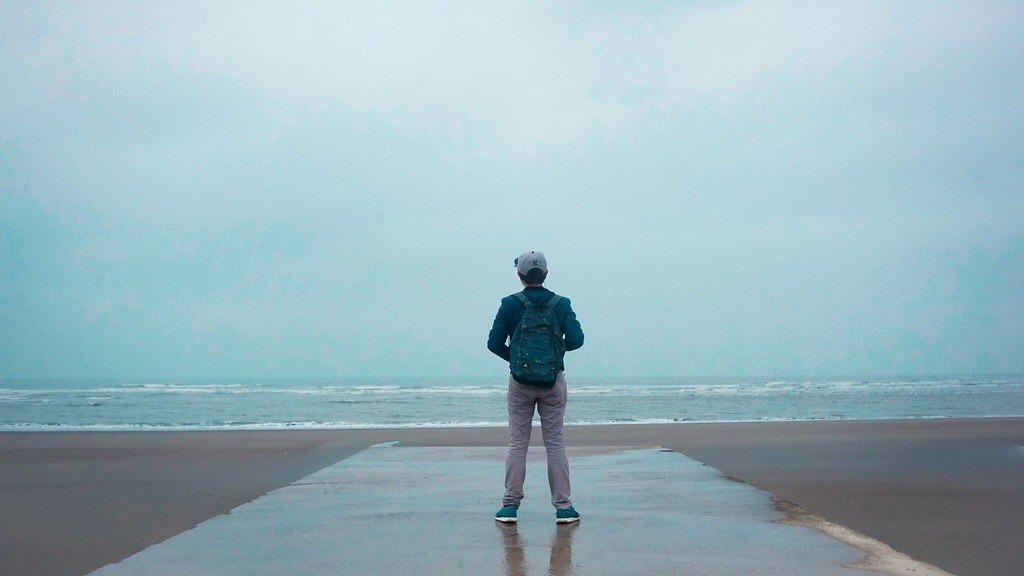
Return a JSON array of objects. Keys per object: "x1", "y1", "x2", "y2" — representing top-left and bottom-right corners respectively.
[{"x1": 514, "y1": 250, "x2": 547, "y2": 275}]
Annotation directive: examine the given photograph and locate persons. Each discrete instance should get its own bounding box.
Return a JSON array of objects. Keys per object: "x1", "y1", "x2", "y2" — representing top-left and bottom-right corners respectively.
[{"x1": 486, "y1": 249, "x2": 584, "y2": 523}]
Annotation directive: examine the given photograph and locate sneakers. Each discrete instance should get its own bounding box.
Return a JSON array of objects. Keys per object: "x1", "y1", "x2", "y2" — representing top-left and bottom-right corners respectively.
[
  {"x1": 495, "y1": 506, "x2": 518, "y2": 523},
  {"x1": 556, "y1": 507, "x2": 580, "y2": 523}
]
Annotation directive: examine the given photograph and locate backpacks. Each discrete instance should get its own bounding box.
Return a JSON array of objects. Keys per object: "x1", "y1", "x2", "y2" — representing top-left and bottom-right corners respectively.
[{"x1": 510, "y1": 291, "x2": 566, "y2": 388}]
